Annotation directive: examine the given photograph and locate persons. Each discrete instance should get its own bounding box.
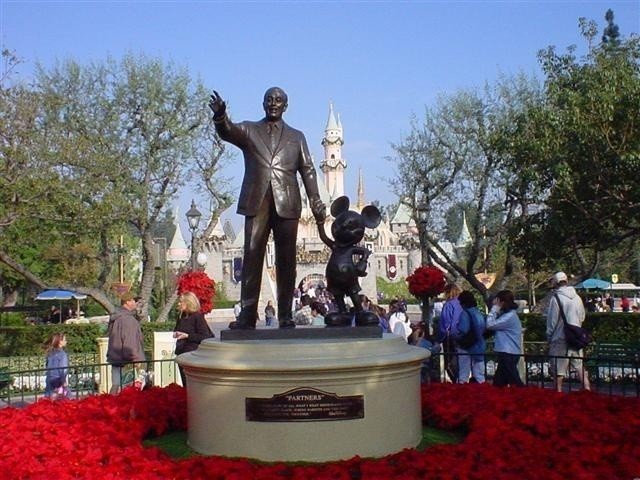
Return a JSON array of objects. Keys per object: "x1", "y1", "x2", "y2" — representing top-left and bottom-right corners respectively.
[
  {"x1": 174, "y1": 292, "x2": 214, "y2": 387},
  {"x1": 48, "y1": 376, "x2": 71, "y2": 402},
  {"x1": 264, "y1": 301, "x2": 276, "y2": 326},
  {"x1": 25, "y1": 300, "x2": 87, "y2": 327},
  {"x1": 233, "y1": 301, "x2": 242, "y2": 320},
  {"x1": 291, "y1": 275, "x2": 386, "y2": 332},
  {"x1": 452, "y1": 288, "x2": 486, "y2": 386},
  {"x1": 437, "y1": 285, "x2": 467, "y2": 381},
  {"x1": 385, "y1": 293, "x2": 442, "y2": 383},
  {"x1": 576, "y1": 288, "x2": 640, "y2": 313},
  {"x1": 485, "y1": 289, "x2": 525, "y2": 387},
  {"x1": 544, "y1": 272, "x2": 592, "y2": 391},
  {"x1": 42, "y1": 333, "x2": 71, "y2": 399},
  {"x1": 107, "y1": 293, "x2": 144, "y2": 393},
  {"x1": 207, "y1": 87, "x2": 326, "y2": 328}
]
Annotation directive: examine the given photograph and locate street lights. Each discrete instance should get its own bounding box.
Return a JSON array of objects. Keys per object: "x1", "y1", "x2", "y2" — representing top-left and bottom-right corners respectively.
[
  {"x1": 185, "y1": 198, "x2": 201, "y2": 270},
  {"x1": 417, "y1": 193, "x2": 432, "y2": 267}
]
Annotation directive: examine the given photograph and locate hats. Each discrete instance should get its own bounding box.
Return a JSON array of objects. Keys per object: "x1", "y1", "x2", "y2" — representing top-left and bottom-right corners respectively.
[
  {"x1": 555, "y1": 272, "x2": 568, "y2": 283},
  {"x1": 122, "y1": 292, "x2": 142, "y2": 301}
]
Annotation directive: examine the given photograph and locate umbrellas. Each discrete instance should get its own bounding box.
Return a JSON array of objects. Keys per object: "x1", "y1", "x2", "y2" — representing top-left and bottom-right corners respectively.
[
  {"x1": 574, "y1": 278, "x2": 610, "y2": 294},
  {"x1": 34, "y1": 289, "x2": 87, "y2": 324}
]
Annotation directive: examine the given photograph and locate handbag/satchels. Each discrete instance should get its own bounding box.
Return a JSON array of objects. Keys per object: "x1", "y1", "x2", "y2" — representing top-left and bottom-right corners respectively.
[
  {"x1": 564, "y1": 324, "x2": 591, "y2": 347},
  {"x1": 457, "y1": 329, "x2": 476, "y2": 349}
]
